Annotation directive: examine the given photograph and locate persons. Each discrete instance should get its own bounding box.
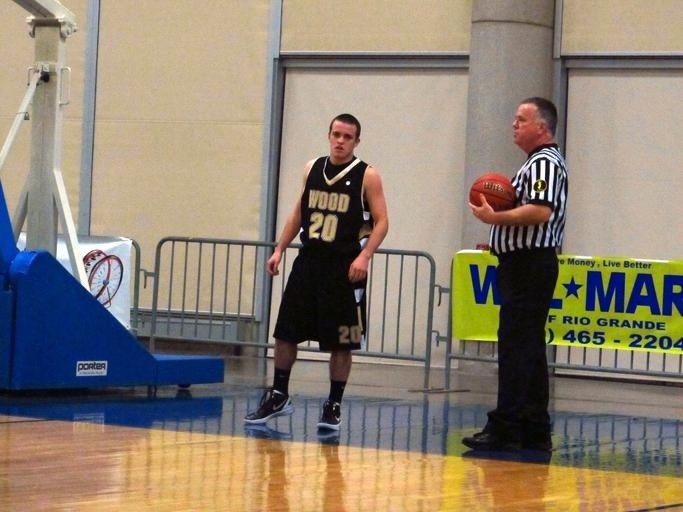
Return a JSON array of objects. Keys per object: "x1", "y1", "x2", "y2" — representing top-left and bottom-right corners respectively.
[
  {"x1": 244, "y1": 113, "x2": 388, "y2": 430},
  {"x1": 463, "y1": 97, "x2": 568, "y2": 452}
]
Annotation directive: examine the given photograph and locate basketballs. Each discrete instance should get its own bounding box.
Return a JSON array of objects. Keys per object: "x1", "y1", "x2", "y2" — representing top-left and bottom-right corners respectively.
[{"x1": 468, "y1": 174, "x2": 517, "y2": 214}]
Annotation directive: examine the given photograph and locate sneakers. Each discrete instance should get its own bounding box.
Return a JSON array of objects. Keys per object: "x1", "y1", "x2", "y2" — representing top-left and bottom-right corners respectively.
[
  {"x1": 243, "y1": 387, "x2": 295, "y2": 424},
  {"x1": 463, "y1": 425, "x2": 551, "y2": 450},
  {"x1": 316, "y1": 399, "x2": 343, "y2": 431}
]
